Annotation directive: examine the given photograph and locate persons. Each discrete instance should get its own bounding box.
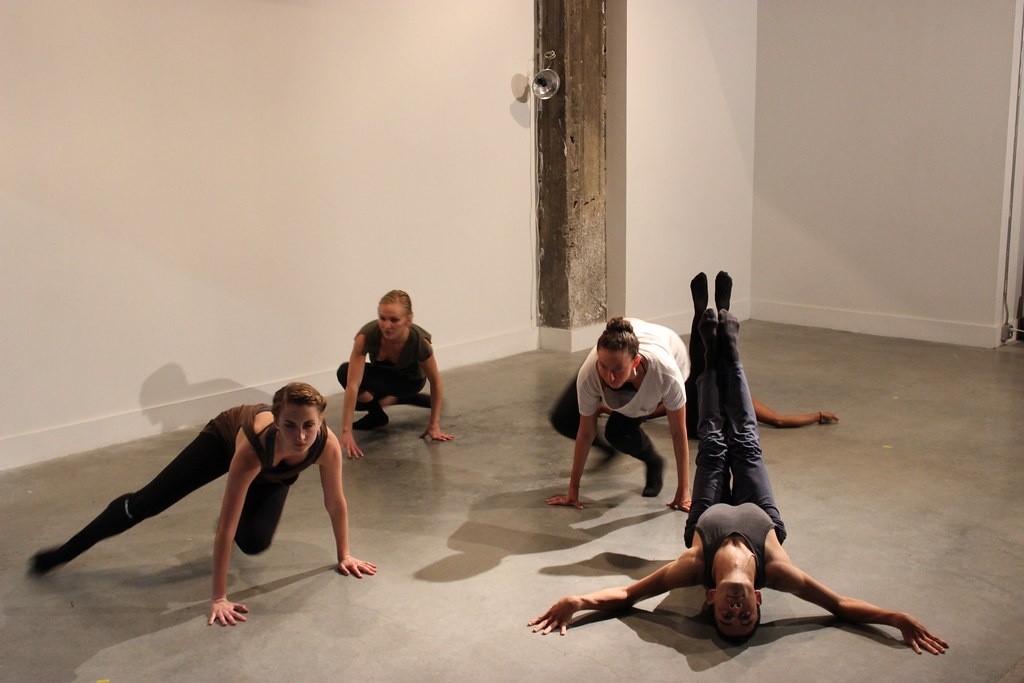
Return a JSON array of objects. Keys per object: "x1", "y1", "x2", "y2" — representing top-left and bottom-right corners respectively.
[
  {"x1": 588, "y1": 270, "x2": 840, "y2": 430},
  {"x1": 336, "y1": 289, "x2": 454, "y2": 460},
  {"x1": 26, "y1": 381, "x2": 379, "y2": 627},
  {"x1": 526, "y1": 308, "x2": 951, "y2": 655}
]
